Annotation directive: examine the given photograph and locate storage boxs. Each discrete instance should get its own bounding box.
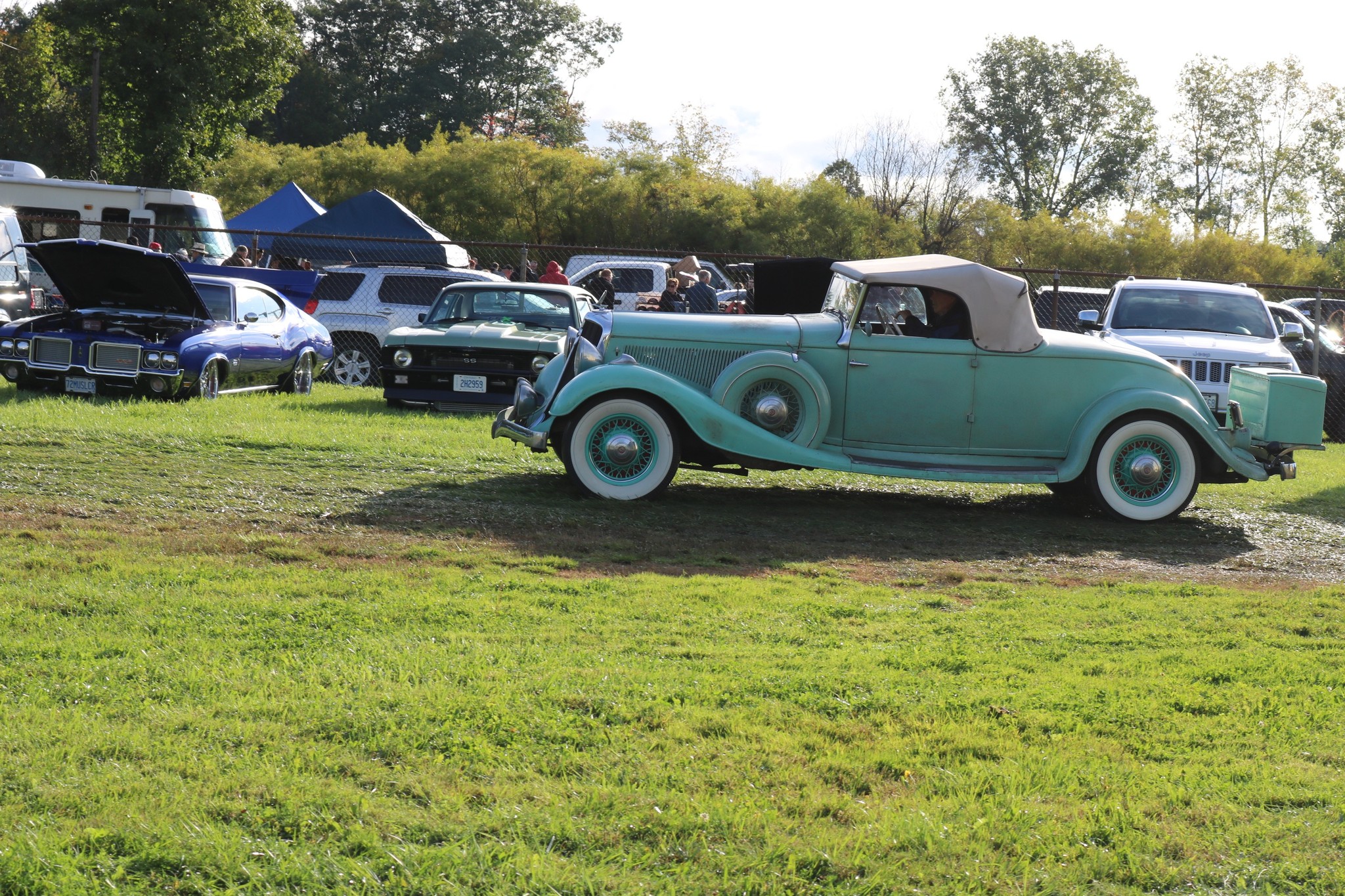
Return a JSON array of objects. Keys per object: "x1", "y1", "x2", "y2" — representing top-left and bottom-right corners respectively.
[{"x1": 1225, "y1": 365, "x2": 1327, "y2": 445}]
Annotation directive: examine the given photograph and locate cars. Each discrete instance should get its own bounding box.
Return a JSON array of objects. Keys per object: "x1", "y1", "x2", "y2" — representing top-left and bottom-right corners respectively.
[
  {"x1": 1033, "y1": 286, "x2": 1344, "y2": 440},
  {"x1": 378, "y1": 283, "x2": 602, "y2": 415},
  {"x1": 2, "y1": 237, "x2": 335, "y2": 403},
  {"x1": 490, "y1": 253, "x2": 1326, "y2": 528}
]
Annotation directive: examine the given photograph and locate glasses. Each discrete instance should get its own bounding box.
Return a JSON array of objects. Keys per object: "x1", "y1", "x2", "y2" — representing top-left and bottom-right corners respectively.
[
  {"x1": 190, "y1": 250, "x2": 196, "y2": 253},
  {"x1": 667, "y1": 285, "x2": 676, "y2": 288}
]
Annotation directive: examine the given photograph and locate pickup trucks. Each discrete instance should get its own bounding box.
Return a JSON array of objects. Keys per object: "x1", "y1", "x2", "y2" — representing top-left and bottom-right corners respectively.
[{"x1": 568, "y1": 262, "x2": 745, "y2": 312}]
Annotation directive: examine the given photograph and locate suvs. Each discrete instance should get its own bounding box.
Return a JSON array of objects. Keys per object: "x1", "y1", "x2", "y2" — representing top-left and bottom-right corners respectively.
[
  {"x1": 1075, "y1": 277, "x2": 1303, "y2": 426},
  {"x1": 305, "y1": 262, "x2": 563, "y2": 387}
]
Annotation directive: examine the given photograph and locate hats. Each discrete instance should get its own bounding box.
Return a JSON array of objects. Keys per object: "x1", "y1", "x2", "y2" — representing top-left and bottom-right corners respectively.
[
  {"x1": 188, "y1": 242, "x2": 209, "y2": 255},
  {"x1": 502, "y1": 264, "x2": 514, "y2": 272},
  {"x1": 149, "y1": 242, "x2": 161, "y2": 250},
  {"x1": 270, "y1": 253, "x2": 282, "y2": 263}
]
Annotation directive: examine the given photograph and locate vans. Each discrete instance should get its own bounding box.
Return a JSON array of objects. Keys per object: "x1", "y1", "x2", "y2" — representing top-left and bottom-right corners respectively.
[
  {"x1": 0, "y1": 207, "x2": 31, "y2": 329},
  {"x1": 566, "y1": 255, "x2": 733, "y2": 298}
]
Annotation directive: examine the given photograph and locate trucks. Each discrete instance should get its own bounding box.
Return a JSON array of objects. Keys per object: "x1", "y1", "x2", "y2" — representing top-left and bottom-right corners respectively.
[{"x1": 0, "y1": 159, "x2": 235, "y2": 295}]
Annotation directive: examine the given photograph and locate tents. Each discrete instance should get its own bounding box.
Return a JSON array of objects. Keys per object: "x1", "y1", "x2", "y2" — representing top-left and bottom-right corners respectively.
[
  {"x1": 226, "y1": 181, "x2": 331, "y2": 264},
  {"x1": 274, "y1": 188, "x2": 470, "y2": 268}
]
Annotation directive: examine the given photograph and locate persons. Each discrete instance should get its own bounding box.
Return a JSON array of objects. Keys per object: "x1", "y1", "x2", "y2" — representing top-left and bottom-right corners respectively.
[
  {"x1": 586, "y1": 268, "x2": 615, "y2": 310},
  {"x1": 733, "y1": 282, "x2": 744, "y2": 289},
  {"x1": 466, "y1": 253, "x2": 514, "y2": 281},
  {"x1": 539, "y1": 261, "x2": 569, "y2": 285},
  {"x1": 529, "y1": 261, "x2": 539, "y2": 283},
  {"x1": 893, "y1": 288, "x2": 971, "y2": 341},
  {"x1": 510, "y1": 259, "x2": 538, "y2": 283},
  {"x1": 126, "y1": 234, "x2": 212, "y2": 265},
  {"x1": 684, "y1": 269, "x2": 720, "y2": 313},
  {"x1": 219, "y1": 245, "x2": 305, "y2": 271},
  {"x1": 660, "y1": 277, "x2": 685, "y2": 313}
]
